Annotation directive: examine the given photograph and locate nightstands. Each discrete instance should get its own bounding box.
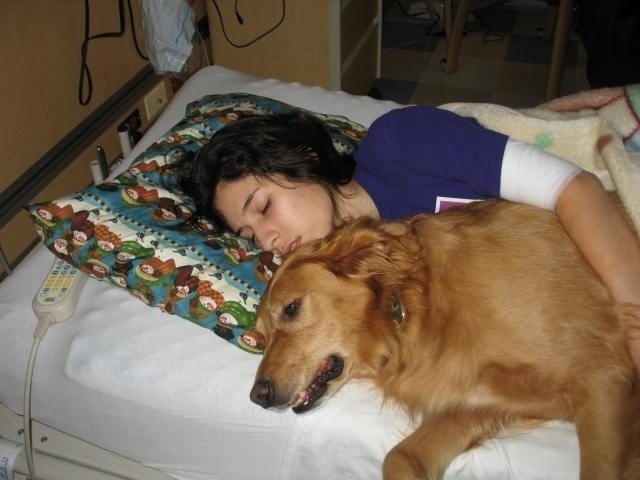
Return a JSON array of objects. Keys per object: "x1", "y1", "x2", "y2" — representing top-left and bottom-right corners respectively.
[{"x1": 208, "y1": 0, "x2": 381, "y2": 93}]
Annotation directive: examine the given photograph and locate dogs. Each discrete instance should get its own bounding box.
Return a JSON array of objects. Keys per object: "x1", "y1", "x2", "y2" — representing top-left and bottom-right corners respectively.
[{"x1": 249, "y1": 190, "x2": 640, "y2": 480}]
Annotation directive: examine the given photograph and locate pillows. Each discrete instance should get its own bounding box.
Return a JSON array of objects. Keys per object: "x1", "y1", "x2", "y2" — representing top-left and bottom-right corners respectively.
[{"x1": 25, "y1": 94, "x2": 367, "y2": 354}]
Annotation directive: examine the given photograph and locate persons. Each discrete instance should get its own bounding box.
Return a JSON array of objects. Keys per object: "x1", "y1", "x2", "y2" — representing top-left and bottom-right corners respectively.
[{"x1": 185, "y1": 104, "x2": 640, "y2": 373}]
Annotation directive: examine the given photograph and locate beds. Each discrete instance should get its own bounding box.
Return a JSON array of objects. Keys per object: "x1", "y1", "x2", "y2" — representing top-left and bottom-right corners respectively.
[{"x1": 2, "y1": 56, "x2": 640, "y2": 480}]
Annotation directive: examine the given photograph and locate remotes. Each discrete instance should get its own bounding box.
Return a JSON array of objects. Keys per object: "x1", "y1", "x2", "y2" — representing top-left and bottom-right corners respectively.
[{"x1": 33, "y1": 258, "x2": 91, "y2": 322}]
